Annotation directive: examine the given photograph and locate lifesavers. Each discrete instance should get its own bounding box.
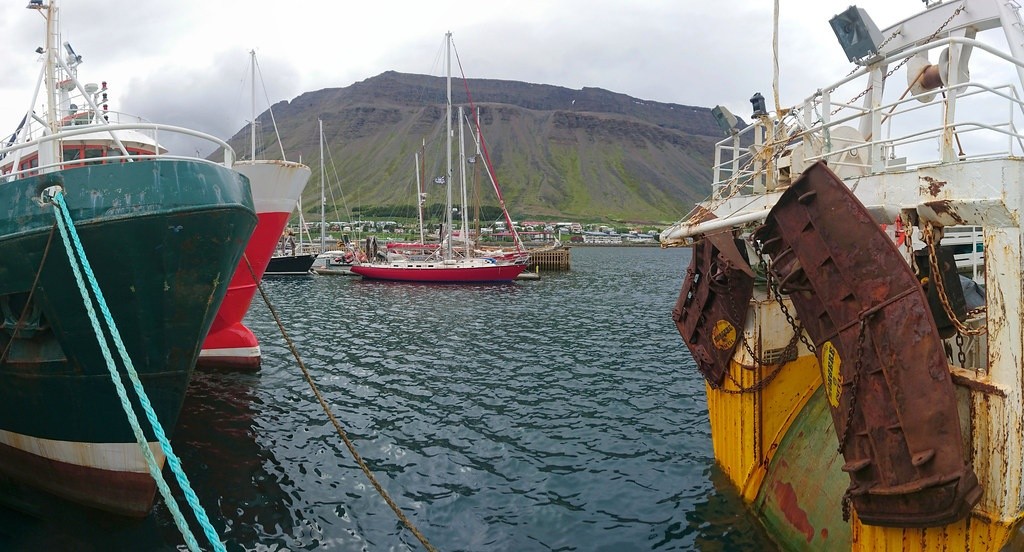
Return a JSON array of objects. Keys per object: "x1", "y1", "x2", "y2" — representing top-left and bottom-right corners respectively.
[{"x1": 880, "y1": 216, "x2": 907, "y2": 250}]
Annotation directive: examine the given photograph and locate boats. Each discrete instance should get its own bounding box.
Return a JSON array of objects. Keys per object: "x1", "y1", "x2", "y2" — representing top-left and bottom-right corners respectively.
[
  {"x1": 656, "y1": 0, "x2": 1024, "y2": 552},
  {"x1": 1, "y1": 1, "x2": 534, "y2": 520}
]
